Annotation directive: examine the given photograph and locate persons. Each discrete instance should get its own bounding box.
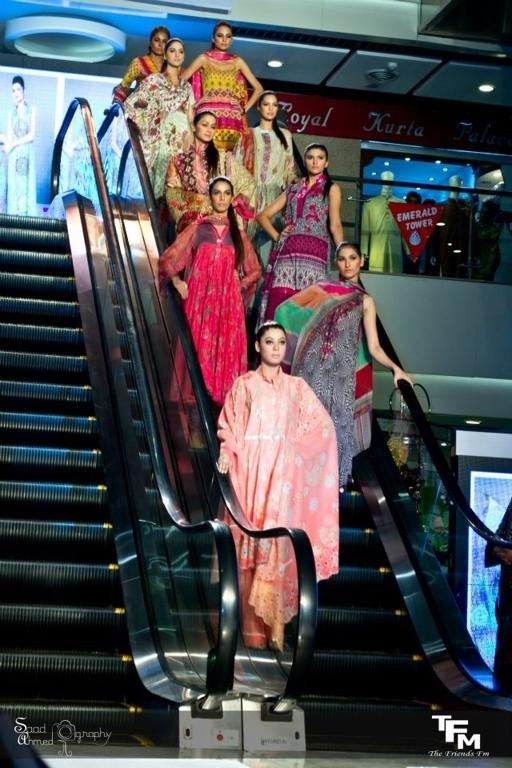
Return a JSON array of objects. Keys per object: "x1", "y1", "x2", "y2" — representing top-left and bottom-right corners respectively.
[
  {"x1": 482, "y1": 498, "x2": 510, "y2": 697},
  {"x1": 213, "y1": 317, "x2": 343, "y2": 657},
  {"x1": 112, "y1": 18, "x2": 301, "y2": 244},
  {"x1": 266, "y1": 239, "x2": 417, "y2": 501},
  {"x1": 256, "y1": 141, "x2": 348, "y2": 328},
  {"x1": 466, "y1": 166, "x2": 511, "y2": 282},
  {"x1": 360, "y1": 168, "x2": 419, "y2": 275},
  {"x1": 402, "y1": 191, "x2": 427, "y2": 275},
  {"x1": 422, "y1": 197, "x2": 443, "y2": 277},
  {"x1": 155, "y1": 173, "x2": 268, "y2": 453},
  {"x1": 426, "y1": 175, "x2": 478, "y2": 280},
  {"x1": 2, "y1": 72, "x2": 43, "y2": 219}
]
